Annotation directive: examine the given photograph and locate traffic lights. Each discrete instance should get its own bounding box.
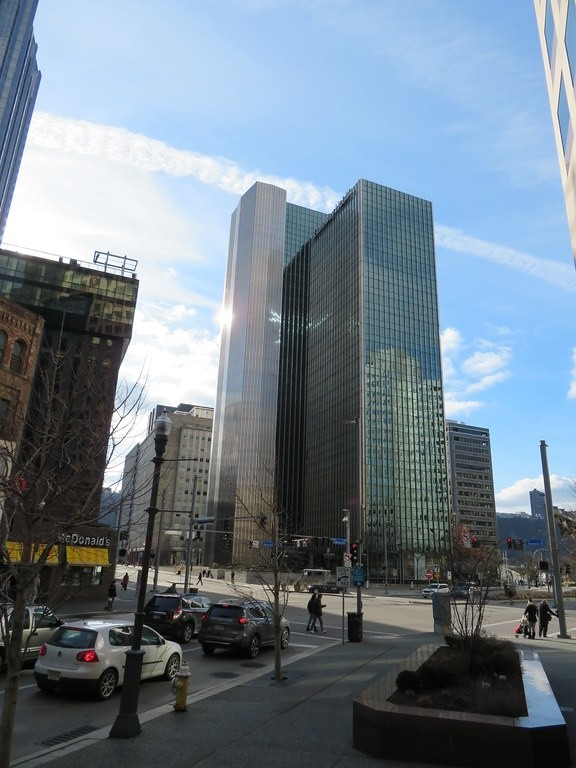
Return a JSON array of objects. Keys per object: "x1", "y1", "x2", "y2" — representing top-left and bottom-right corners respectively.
[
  {"x1": 507, "y1": 537, "x2": 512, "y2": 549},
  {"x1": 260, "y1": 514, "x2": 268, "y2": 524},
  {"x1": 120, "y1": 530, "x2": 129, "y2": 541},
  {"x1": 350, "y1": 543, "x2": 362, "y2": 563},
  {"x1": 564, "y1": 563, "x2": 571, "y2": 573},
  {"x1": 472, "y1": 536, "x2": 477, "y2": 547}
]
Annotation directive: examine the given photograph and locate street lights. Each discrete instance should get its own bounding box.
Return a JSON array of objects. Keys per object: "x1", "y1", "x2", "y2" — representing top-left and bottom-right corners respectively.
[{"x1": 108, "y1": 407, "x2": 174, "y2": 739}]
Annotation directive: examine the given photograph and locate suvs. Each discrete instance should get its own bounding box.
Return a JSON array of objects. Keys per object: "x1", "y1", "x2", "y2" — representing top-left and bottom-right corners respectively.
[
  {"x1": 198, "y1": 598, "x2": 291, "y2": 660},
  {"x1": 143, "y1": 592, "x2": 214, "y2": 643}
]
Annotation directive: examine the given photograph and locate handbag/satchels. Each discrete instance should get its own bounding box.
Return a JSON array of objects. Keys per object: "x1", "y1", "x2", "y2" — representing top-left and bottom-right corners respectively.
[{"x1": 546, "y1": 613, "x2": 552, "y2": 621}]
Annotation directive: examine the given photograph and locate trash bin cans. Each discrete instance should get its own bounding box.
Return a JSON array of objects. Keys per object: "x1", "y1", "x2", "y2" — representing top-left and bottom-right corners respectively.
[
  {"x1": 346, "y1": 612, "x2": 364, "y2": 642},
  {"x1": 505, "y1": 584, "x2": 516, "y2": 597}
]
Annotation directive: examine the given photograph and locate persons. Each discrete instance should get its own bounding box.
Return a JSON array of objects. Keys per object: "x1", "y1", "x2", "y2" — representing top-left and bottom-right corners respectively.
[
  {"x1": 231, "y1": 572, "x2": 235, "y2": 583},
  {"x1": 196, "y1": 572, "x2": 203, "y2": 585},
  {"x1": 523, "y1": 598, "x2": 537, "y2": 639},
  {"x1": 307, "y1": 594, "x2": 327, "y2": 633},
  {"x1": 107, "y1": 581, "x2": 116, "y2": 608},
  {"x1": 167, "y1": 583, "x2": 176, "y2": 592},
  {"x1": 178, "y1": 562, "x2": 182, "y2": 574},
  {"x1": 123, "y1": 573, "x2": 129, "y2": 590},
  {"x1": 203, "y1": 570, "x2": 211, "y2": 577},
  {"x1": 537, "y1": 600, "x2": 557, "y2": 637},
  {"x1": 508, "y1": 589, "x2": 514, "y2": 605}
]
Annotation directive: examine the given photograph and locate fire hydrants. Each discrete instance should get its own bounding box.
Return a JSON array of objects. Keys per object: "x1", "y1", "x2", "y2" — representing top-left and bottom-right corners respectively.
[{"x1": 170, "y1": 660, "x2": 192, "y2": 713}]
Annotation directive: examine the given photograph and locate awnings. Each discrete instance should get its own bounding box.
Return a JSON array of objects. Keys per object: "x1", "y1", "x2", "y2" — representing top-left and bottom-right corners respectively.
[{"x1": 3, "y1": 542, "x2": 110, "y2": 568}]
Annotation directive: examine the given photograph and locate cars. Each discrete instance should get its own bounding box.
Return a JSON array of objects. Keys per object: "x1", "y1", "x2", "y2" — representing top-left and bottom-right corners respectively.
[
  {"x1": 422, "y1": 582, "x2": 454, "y2": 599},
  {"x1": 0, "y1": 602, "x2": 79, "y2": 670},
  {"x1": 300, "y1": 580, "x2": 346, "y2": 594},
  {"x1": 33, "y1": 618, "x2": 183, "y2": 701},
  {"x1": 454, "y1": 581, "x2": 479, "y2": 599}
]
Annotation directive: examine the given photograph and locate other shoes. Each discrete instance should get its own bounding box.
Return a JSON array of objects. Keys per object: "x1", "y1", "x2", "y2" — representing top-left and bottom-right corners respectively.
[
  {"x1": 543, "y1": 634, "x2": 547, "y2": 637},
  {"x1": 314, "y1": 630, "x2": 318, "y2": 632},
  {"x1": 306, "y1": 628, "x2": 309, "y2": 631},
  {"x1": 322, "y1": 630, "x2": 328, "y2": 633},
  {"x1": 527, "y1": 636, "x2": 535, "y2": 639},
  {"x1": 539, "y1": 632, "x2": 541, "y2": 637},
  {"x1": 311, "y1": 630, "x2": 315, "y2": 633}
]
